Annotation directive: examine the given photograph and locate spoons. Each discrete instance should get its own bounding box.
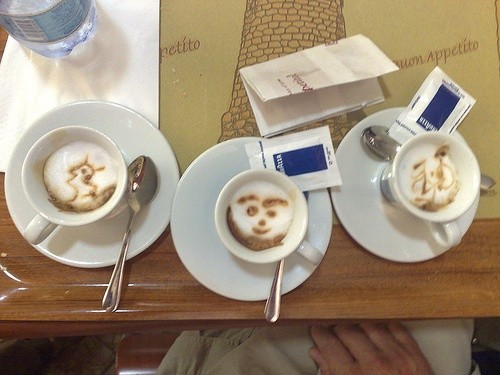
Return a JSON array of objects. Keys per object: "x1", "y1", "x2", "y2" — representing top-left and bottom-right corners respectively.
[
  {"x1": 360, "y1": 125, "x2": 496, "y2": 193},
  {"x1": 101, "y1": 155, "x2": 158, "y2": 313}
]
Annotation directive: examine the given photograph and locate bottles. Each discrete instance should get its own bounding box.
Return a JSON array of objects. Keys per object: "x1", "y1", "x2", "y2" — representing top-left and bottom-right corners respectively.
[{"x1": 0, "y1": 0, "x2": 98, "y2": 60}]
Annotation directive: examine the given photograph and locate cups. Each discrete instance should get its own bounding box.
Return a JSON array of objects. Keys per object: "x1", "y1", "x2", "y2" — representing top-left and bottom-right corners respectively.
[
  {"x1": 214, "y1": 167, "x2": 323, "y2": 266},
  {"x1": 380, "y1": 132, "x2": 481, "y2": 248},
  {"x1": 21, "y1": 125, "x2": 129, "y2": 246}
]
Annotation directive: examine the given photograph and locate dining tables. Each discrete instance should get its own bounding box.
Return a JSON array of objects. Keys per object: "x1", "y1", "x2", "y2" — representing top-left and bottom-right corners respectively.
[{"x1": 0, "y1": 0, "x2": 500, "y2": 323}]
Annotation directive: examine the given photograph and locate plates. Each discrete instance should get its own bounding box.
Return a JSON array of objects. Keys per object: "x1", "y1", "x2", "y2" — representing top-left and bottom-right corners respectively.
[
  {"x1": 3, "y1": 99, "x2": 181, "y2": 268},
  {"x1": 171, "y1": 137, "x2": 332, "y2": 301},
  {"x1": 330, "y1": 106, "x2": 481, "y2": 263}
]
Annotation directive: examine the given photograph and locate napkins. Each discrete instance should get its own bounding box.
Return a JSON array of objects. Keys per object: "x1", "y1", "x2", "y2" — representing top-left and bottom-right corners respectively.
[{"x1": 0, "y1": 0, "x2": 161, "y2": 173}]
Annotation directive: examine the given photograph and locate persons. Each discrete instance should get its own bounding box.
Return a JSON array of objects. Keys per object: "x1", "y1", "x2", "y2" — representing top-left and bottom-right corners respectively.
[{"x1": 156, "y1": 319, "x2": 476, "y2": 375}]
